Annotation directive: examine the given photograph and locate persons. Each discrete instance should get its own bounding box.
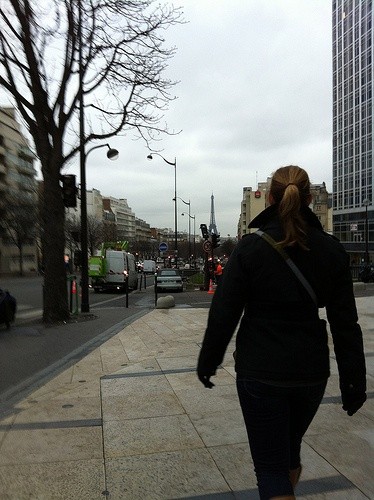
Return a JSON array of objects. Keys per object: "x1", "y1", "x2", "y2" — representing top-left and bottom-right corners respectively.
[
  {"x1": 214, "y1": 262, "x2": 222, "y2": 284},
  {"x1": 196, "y1": 165, "x2": 367, "y2": 500}
]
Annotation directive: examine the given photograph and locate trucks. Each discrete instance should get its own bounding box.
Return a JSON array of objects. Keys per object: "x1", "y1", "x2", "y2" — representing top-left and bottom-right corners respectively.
[{"x1": 88, "y1": 240, "x2": 138, "y2": 293}]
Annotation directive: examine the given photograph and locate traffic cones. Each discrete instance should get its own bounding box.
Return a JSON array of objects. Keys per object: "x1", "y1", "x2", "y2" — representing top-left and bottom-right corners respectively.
[{"x1": 207, "y1": 279, "x2": 215, "y2": 294}]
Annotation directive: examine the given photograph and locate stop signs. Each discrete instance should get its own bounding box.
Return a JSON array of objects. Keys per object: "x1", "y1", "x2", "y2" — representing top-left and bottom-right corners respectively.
[{"x1": 73, "y1": 279, "x2": 76, "y2": 294}]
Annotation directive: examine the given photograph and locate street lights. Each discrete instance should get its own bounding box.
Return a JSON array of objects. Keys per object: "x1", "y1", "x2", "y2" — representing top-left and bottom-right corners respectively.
[
  {"x1": 147, "y1": 153, "x2": 178, "y2": 268},
  {"x1": 79, "y1": 83, "x2": 119, "y2": 314},
  {"x1": 181, "y1": 212, "x2": 196, "y2": 268},
  {"x1": 363, "y1": 198, "x2": 371, "y2": 284},
  {"x1": 173, "y1": 197, "x2": 191, "y2": 264}
]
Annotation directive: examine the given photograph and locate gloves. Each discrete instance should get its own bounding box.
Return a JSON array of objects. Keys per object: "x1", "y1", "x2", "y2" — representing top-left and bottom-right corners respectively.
[
  {"x1": 341, "y1": 392, "x2": 367, "y2": 416},
  {"x1": 197, "y1": 375, "x2": 214, "y2": 389}
]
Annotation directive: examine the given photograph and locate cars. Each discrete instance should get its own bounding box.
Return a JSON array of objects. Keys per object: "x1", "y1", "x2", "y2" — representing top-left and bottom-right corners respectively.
[{"x1": 154, "y1": 270, "x2": 184, "y2": 293}]
